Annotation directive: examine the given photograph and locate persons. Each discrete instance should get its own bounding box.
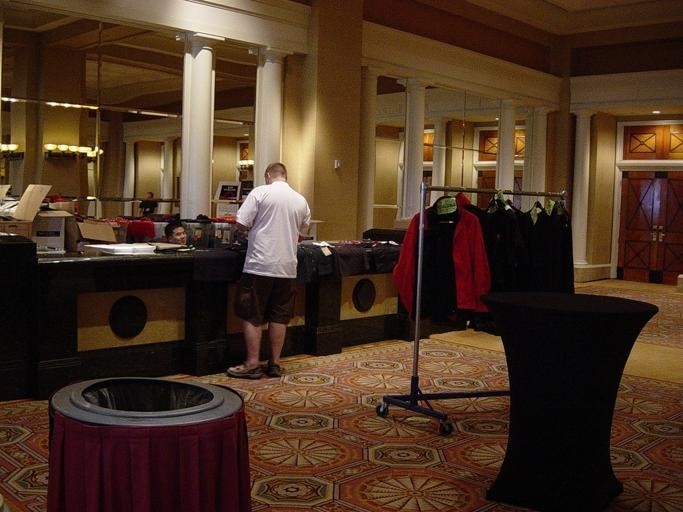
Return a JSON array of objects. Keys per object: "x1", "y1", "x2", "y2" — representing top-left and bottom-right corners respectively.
[
  {"x1": 227, "y1": 162, "x2": 312, "y2": 378},
  {"x1": 138, "y1": 192, "x2": 158, "y2": 217},
  {"x1": 165, "y1": 221, "x2": 187, "y2": 245}
]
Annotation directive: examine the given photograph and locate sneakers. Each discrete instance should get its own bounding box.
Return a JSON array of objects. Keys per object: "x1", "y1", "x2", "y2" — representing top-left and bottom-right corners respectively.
[{"x1": 227, "y1": 360, "x2": 283, "y2": 379}]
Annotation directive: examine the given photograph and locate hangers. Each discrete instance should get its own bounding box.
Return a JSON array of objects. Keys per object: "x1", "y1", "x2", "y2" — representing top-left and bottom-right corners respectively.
[{"x1": 413, "y1": 184, "x2": 569, "y2": 239}]
